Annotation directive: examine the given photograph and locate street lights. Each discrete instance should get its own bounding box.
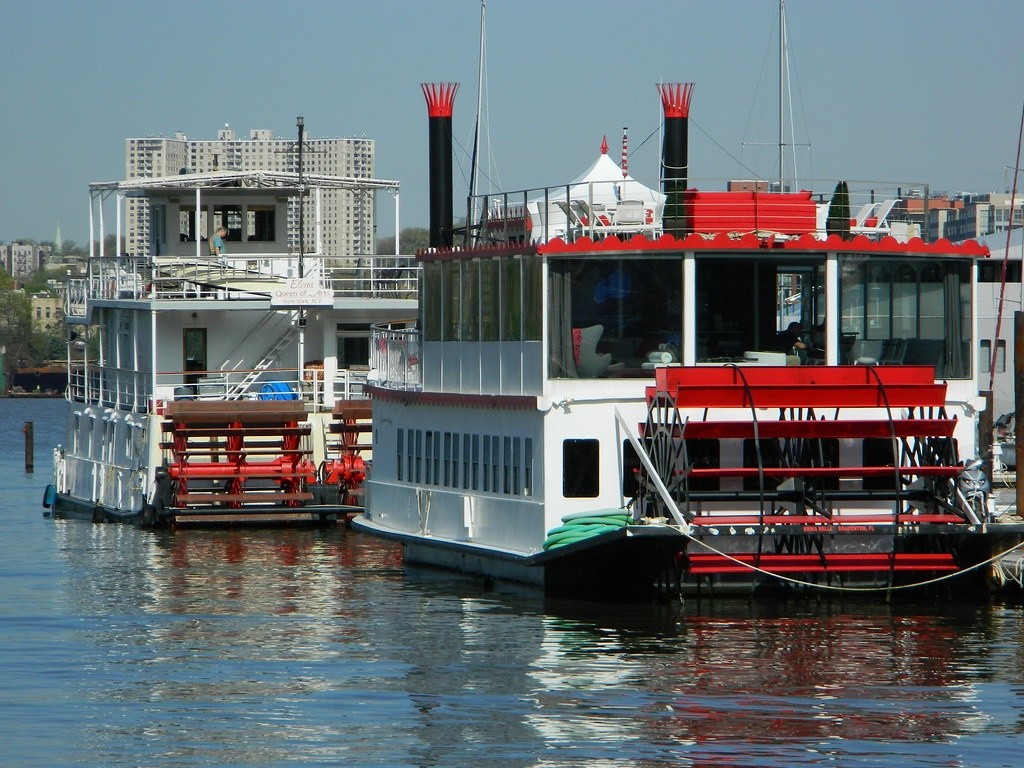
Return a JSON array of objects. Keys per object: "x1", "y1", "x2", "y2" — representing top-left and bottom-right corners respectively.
[{"x1": 295, "y1": 114, "x2": 304, "y2": 276}]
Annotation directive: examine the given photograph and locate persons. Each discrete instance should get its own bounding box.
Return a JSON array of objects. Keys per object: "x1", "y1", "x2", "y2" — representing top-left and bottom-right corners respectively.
[
  {"x1": 776, "y1": 322, "x2": 811, "y2": 365},
  {"x1": 209, "y1": 227, "x2": 229, "y2": 257}
]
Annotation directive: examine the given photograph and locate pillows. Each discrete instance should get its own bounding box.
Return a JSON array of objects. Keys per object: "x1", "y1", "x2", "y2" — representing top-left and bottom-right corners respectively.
[
  {"x1": 864, "y1": 217, "x2": 878, "y2": 227},
  {"x1": 850, "y1": 217, "x2": 856, "y2": 227}
]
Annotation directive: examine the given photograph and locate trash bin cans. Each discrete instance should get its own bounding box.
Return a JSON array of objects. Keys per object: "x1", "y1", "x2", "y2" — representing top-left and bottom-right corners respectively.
[{"x1": 174, "y1": 387, "x2": 193, "y2": 401}]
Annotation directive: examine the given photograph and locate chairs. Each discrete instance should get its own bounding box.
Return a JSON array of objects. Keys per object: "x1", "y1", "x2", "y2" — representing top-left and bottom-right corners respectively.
[
  {"x1": 374, "y1": 264, "x2": 406, "y2": 301},
  {"x1": 786, "y1": 337, "x2": 909, "y2": 366},
  {"x1": 848, "y1": 199, "x2": 902, "y2": 241},
  {"x1": 101, "y1": 262, "x2": 144, "y2": 301},
  {"x1": 572, "y1": 323, "x2": 611, "y2": 378},
  {"x1": 553, "y1": 198, "x2": 664, "y2": 241}
]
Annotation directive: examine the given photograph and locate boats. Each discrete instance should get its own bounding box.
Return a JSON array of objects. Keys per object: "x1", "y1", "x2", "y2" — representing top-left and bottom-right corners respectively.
[
  {"x1": 352, "y1": 80, "x2": 1024, "y2": 604},
  {"x1": 41, "y1": 167, "x2": 422, "y2": 526}
]
